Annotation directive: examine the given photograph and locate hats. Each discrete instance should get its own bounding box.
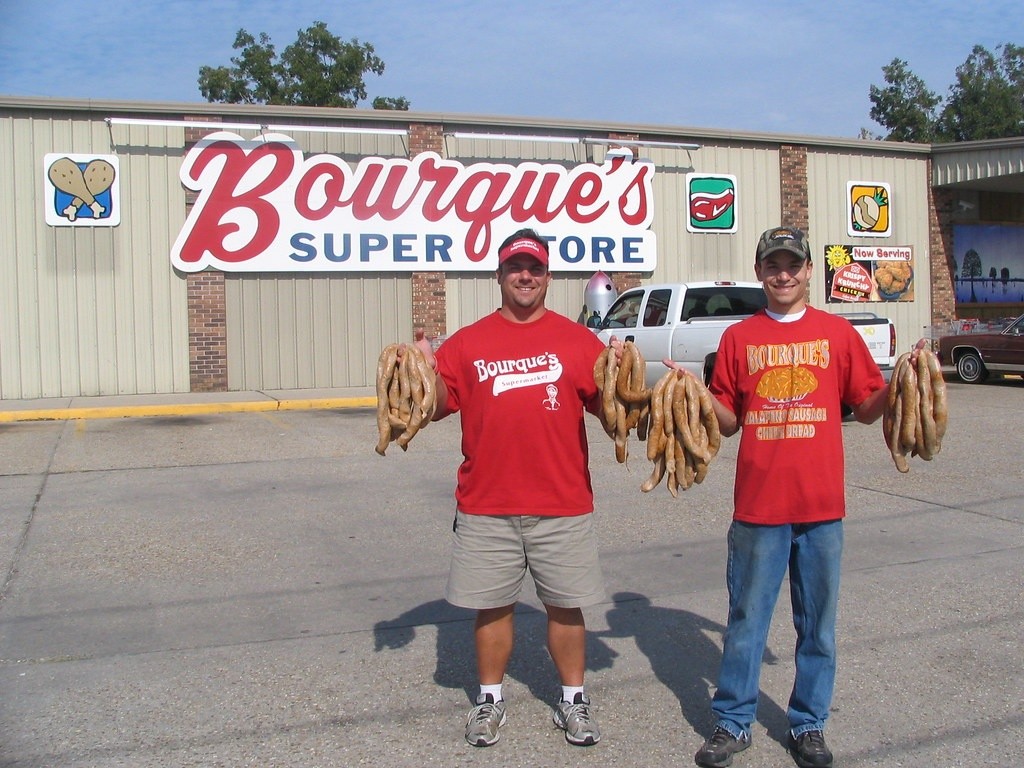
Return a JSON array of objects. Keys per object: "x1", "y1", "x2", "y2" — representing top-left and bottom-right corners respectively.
[
  {"x1": 498, "y1": 237, "x2": 549, "y2": 270},
  {"x1": 755, "y1": 226, "x2": 811, "y2": 263}
]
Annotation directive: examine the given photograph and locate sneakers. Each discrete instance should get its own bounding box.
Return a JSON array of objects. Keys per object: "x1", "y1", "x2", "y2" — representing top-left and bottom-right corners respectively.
[
  {"x1": 694, "y1": 720, "x2": 752, "y2": 768},
  {"x1": 465, "y1": 694, "x2": 507, "y2": 747},
  {"x1": 553, "y1": 692, "x2": 602, "y2": 746},
  {"x1": 783, "y1": 722, "x2": 834, "y2": 768}
]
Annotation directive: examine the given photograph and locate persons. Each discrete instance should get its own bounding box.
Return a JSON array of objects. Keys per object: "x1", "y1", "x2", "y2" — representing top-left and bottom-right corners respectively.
[
  {"x1": 663, "y1": 227, "x2": 925, "y2": 768},
  {"x1": 396, "y1": 228, "x2": 624, "y2": 746}
]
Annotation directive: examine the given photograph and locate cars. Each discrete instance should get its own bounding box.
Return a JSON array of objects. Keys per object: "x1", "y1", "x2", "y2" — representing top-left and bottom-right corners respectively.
[{"x1": 938, "y1": 314, "x2": 1024, "y2": 384}]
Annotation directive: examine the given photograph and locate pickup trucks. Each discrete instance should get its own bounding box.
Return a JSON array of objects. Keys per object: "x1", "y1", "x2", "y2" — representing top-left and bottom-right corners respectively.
[{"x1": 585, "y1": 282, "x2": 897, "y2": 418}]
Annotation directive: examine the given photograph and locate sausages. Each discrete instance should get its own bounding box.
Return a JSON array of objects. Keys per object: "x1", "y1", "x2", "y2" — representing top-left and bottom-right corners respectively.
[
  {"x1": 375, "y1": 343, "x2": 437, "y2": 455},
  {"x1": 883, "y1": 350, "x2": 947, "y2": 472},
  {"x1": 591, "y1": 341, "x2": 720, "y2": 497}
]
